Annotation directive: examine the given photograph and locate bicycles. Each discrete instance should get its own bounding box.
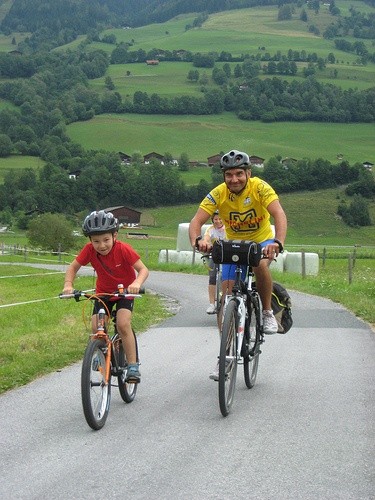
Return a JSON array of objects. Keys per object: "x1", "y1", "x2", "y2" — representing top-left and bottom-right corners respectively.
[
  {"x1": 196, "y1": 246, "x2": 280, "y2": 416},
  {"x1": 58, "y1": 284, "x2": 146, "y2": 431}
]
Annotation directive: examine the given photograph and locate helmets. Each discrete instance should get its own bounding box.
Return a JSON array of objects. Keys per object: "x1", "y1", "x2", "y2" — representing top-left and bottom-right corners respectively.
[
  {"x1": 220, "y1": 150, "x2": 251, "y2": 171},
  {"x1": 81, "y1": 210, "x2": 119, "y2": 237}
]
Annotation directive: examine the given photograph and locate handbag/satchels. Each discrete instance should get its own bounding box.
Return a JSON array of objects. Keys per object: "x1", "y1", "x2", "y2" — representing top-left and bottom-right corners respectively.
[
  {"x1": 252, "y1": 281, "x2": 293, "y2": 334},
  {"x1": 212, "y1": 239, "x2": 261, "y2": 266}
]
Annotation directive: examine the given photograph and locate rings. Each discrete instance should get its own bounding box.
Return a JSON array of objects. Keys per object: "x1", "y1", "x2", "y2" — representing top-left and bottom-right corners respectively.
[{"x1": 272, "y1": 250, "x2": 275, "y2": 252}]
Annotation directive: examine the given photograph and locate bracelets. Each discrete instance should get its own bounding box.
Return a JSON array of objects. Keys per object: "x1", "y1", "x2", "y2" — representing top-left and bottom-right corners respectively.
[{"x1": 64, "y1": 280, "x2": 73, "y2": 284}]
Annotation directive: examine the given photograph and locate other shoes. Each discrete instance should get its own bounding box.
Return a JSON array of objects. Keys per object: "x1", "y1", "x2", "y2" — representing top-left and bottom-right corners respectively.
[{"x1": 207, "y1": 304, "x2": 215, "y2": 314}]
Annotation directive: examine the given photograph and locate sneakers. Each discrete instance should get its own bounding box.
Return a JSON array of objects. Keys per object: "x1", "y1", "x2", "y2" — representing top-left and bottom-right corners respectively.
[
  {"x1": 209, "y1": 362, "x2": 219, "y2": 380},
  {"x1": 124, "y1": 363, "x2": 141, "y2": 381},
  {"x1": 92, "y1": 356, "x2": 99, "y2": 371},
  {"x1": 262, "y1": 310, "x2": 278, "y2": 334}
]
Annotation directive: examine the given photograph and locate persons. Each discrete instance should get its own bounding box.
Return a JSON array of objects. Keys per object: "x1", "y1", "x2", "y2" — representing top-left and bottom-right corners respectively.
[
  {"x1": 189, "y1": 150, "x2": 287, "y2": 379},
  {"x1": 205, "y1": 209, "x2": 227, "y2": 314},
  {"x1": 63, "y1": 210, "x2": 149, "y2": 377}
]
